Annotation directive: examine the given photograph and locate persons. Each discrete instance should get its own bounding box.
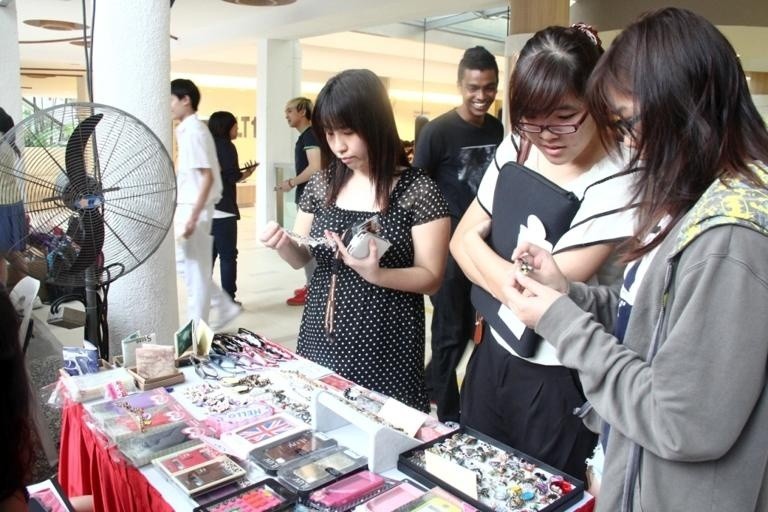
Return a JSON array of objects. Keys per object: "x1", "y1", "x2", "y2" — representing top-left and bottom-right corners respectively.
[
  {"x1": 272, "y1": 96, "x2": 331, "y2": 308},
  {"x1": 410, "y1": 44, "x2": 503, "y2": 424},
  {"x1": 0, "y1": 285, "x2": 43, "y2": 512},
  {"x1": 446, "y1": 16, "x2": 654, "y2": 492},
  {"x1": 166, "y1": 76, "x2": 242, "y2": 343},
  {"x1": 505, "y1": 10, "x2": 767, "y2": 512},
  {"x1": 263, "y1": 67, "x2": 453, "y2": 414},
  {"x1": 0, "y1": 108, "x2": 33, "y2": 288},
  {"x1": 207, "y1": 108, "x2": 260, "y2": 304}
]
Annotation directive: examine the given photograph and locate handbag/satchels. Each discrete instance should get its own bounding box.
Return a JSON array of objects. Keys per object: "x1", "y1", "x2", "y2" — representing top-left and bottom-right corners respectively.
[{"x1": 469, "y1": 160, "x2": 579, "y2": 358}]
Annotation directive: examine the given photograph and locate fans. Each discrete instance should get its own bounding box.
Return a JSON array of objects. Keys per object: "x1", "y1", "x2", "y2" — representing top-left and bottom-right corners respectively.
[{"x1": 0, "y1": 102, "x2": 178, "y2": 365}]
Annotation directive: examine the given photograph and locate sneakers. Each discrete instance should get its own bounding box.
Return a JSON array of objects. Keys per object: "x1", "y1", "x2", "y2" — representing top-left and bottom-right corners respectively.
[{"x1": 287, "y1": 285, "x2": 307, "y2": 306}]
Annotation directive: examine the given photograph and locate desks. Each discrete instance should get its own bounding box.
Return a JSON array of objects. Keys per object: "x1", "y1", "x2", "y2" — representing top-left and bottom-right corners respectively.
[{"x1": 53, "y1": 332, "x2": 596, "y2": 512}]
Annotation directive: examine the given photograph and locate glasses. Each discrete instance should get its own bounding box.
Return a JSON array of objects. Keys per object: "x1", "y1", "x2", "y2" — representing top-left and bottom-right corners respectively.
[
  {"x1": 611, "y1": 114, "x2": 645, "y2": 136},
  {"x1": 512, "y1": 104, "x2": 591, "y2": 137},
  {"x1": 188, "y1": 329, "x2": 295, "y2": 381}
]
